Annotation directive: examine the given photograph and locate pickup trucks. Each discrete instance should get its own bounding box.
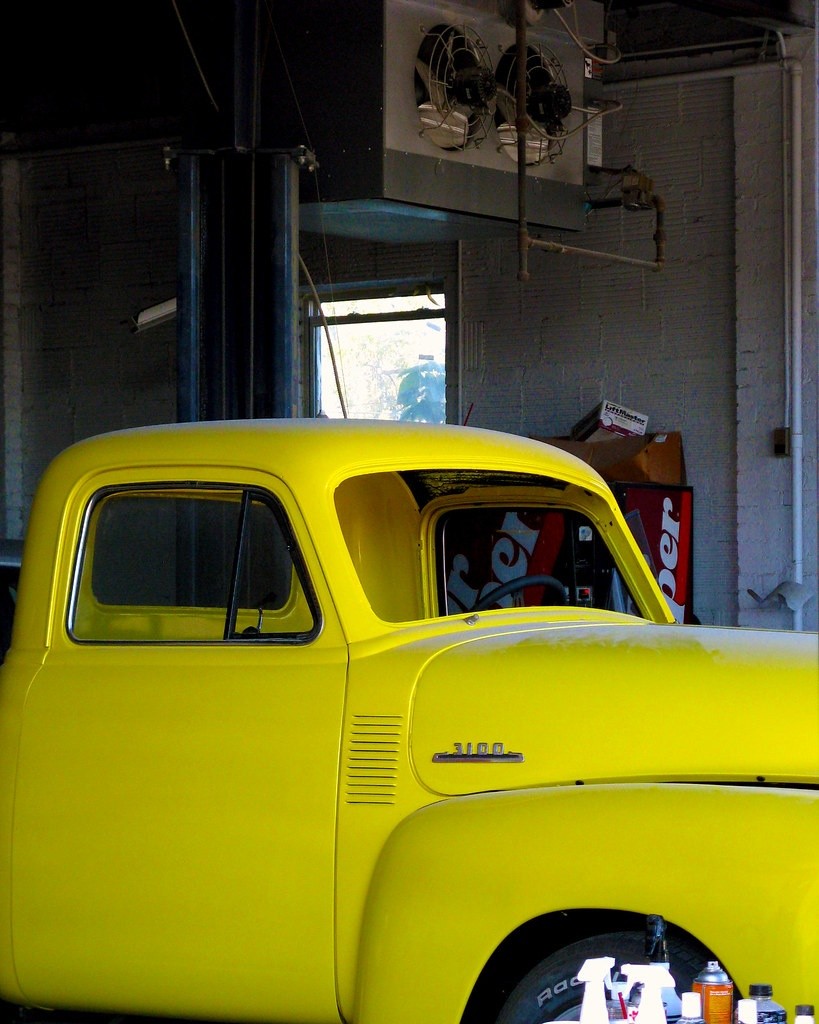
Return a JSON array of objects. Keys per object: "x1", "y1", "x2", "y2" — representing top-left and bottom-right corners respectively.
[{"x1": 0, "y1": 419, "x2": 819, "y2": 1024}]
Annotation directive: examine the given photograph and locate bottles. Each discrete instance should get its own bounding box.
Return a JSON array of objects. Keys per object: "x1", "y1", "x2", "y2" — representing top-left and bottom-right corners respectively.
[{"x1": 577, "y1": 912, "x2": 814, "y2": 1024}]
